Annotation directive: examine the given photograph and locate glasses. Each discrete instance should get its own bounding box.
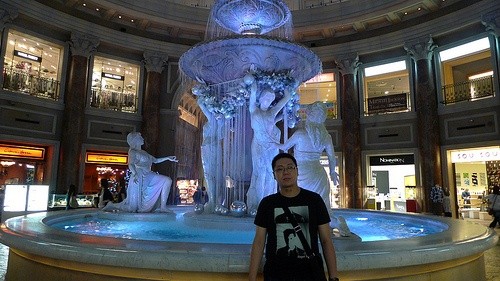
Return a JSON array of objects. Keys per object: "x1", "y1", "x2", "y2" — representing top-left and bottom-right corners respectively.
[{"x1": 273, "y1": 166, "x2": 297, "y2": 173}]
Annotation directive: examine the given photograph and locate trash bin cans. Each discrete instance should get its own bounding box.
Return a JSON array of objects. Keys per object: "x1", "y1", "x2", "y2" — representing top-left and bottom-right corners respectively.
[{"x1": 406, "y1": 199, "x2": 416, "y2": 213}]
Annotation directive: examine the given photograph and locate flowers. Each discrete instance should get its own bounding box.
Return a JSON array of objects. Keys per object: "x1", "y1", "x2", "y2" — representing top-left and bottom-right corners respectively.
[{"x1": 429, "y1": 184, "x2": 444, "y2": 202}]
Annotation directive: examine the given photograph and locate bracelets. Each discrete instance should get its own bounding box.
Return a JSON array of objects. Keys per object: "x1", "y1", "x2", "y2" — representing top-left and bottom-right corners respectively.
[{"x1": 328, "y1": 277, "x2": 339, "y2": 281}]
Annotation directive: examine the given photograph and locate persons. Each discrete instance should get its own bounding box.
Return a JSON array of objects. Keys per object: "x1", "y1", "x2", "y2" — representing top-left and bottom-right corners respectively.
[
  {"x1": 277, "y1": 229, "x2": 306, "y2": 258},
  {"x1": 101, "y1": 131, "x2": 180, "y2": 213},
  {"x1": 266, "y1": 99, "x2": 341, "y2": 239},
  {"x1": 460, "y1": 188, "x2": 471, "y2": 208},
  {"x1": 482, "y1": 186, "x2": 500, "y2": 229},
  {"x1": 66, "y1": 182, "x2": 78, "y2": 212},
  {"x1": 247, "y1": 83, "x2": 291, "y2": 210},
  {"x1": 98, "y1": 174, "x2": 127, "y2": 210},
  {"x1": 196, "y1": 93, "x2": 223, "y2": 210},
  {"x1": 247, "y1": 152, "x2": 340, "y2": 281},
  {"x1": 429, "y1": 184, "x2": 453, "y2": 218}
]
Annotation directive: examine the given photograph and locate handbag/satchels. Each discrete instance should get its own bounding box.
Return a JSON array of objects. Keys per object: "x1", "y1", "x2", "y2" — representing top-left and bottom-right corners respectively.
[
  {"x1": 304, "y1": 250, "x2": 327, "y2": 281},
  {"x1": 489, "y1": 208, "x2": 495, "y2": 216}
]
